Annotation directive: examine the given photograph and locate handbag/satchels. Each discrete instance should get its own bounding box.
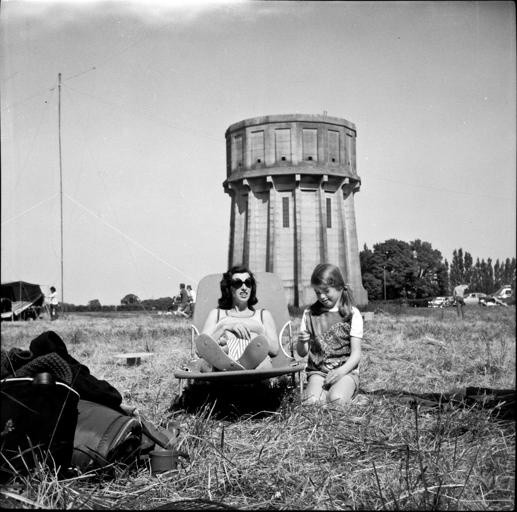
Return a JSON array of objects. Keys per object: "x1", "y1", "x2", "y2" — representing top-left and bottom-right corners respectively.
[{"x1": 1, "y1": 373, "x2": 81, "y2": 482}]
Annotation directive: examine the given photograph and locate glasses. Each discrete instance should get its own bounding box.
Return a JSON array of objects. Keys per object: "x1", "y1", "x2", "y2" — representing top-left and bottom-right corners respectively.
[{"x1": 234, "y1": 277, "x2": 253, "y2": 289}]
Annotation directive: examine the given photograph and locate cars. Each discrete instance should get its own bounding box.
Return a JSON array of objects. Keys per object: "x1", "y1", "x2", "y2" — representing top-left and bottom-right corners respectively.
[{"x1": 428, "y1": 284, "x2": 513, "y2": 307}]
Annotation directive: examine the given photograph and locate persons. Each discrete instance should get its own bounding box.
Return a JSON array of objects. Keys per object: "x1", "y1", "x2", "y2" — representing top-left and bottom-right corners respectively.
[
  {"x1": 185, "y1": 285, "x2": 196, "y2": 318},
  {"x1": 453, "y1": 280, "x2": 471, "y2": 319},
  {"x1": 193, "y1": 264, "x2": 280, "y2": 373},
  {"x1": 173, "y1": 283, "x2": 193, "y2": 319},
  {"x1": 44, "y1": 286, "x2": 59, "y2": 319},
  {"x1": 296, "y1": 263, "x2": 369, "y2": 408}
]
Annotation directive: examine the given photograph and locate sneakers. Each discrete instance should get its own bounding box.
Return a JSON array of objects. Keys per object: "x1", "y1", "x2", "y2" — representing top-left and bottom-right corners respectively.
[
  {"x1": 235, "y1": 335, "x2": 269, "y2": 370},
  {"x1": 197, "y1": 334, "x2": 245, "y2": 371}
]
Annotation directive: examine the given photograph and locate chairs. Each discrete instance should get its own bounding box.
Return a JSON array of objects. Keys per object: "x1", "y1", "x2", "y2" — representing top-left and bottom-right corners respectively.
[{"x1": 173, "y1": 272, "x2": 305, "y2": 404}]
